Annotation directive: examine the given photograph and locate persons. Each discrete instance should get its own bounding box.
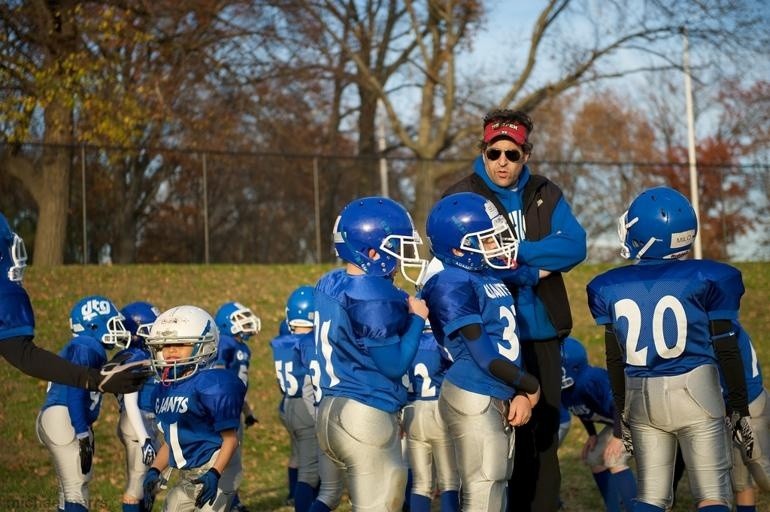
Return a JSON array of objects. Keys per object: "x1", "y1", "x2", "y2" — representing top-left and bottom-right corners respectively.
[
  {"x1": 674, "y1": 325, "x2": 769, "y2": 512},
  {"x1": 268, "y1": 285, "x2": 344, "y2": 512},
  {"x1": 35, "y1": 295, "x2": 262, "y2": 512},
  {"x1": 1, "y1": 213, "x2": 155, "y2": 396},
  {"x1": 408, "y1": 192, "x2": 540, "y2": 510},
  {"x1": 587, "y1": 185, "x2": 755, "y2": 510},
  {"x1": 438, "y1": 106, "x2": 587, "y2": 511},
  {"x1": 400, "y1": 292, "x2": 465, "y2": 512},
  {"x1": 311, "y1": 194, "x2": 429, "y2": 510},
  {"x1": 559, "y1": 339, "x2": 638, "y2": 510}
]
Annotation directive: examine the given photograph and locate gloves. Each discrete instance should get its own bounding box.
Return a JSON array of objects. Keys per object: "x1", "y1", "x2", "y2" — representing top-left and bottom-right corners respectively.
[
  {"x1": 79, "y1": 354, "x2": 156, "y2": 394},
  {"x1": 620, "y1": 412, "x2": 634, "y2": 456},
  {"x1": 79, "y1": 435, "x2": 92, "y2": 475},
  {"x1": 728, "y1": 408, "x2": 754, "y2": 460},
  {"x1": 189, "y1": 467, "x2": 221, "y2": 509},
  {"x1": 142, "y1": 467, "x2": 166, "y2": 511},
  {"x1": 244, "y1": 415, "x2": 259, "y2": 426},
  {"x1": 141, "y1": 438, "x2": 159, "y2": 465}
]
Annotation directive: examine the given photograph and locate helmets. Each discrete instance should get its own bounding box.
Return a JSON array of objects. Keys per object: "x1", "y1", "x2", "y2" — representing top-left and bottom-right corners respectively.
[
  {"x1": 561, "y1": 338, "x2": 589, "y2": 390},
  {"x1": 425, "y1": 191, "x2": 520, "y2": 271},
  {"x1": 332, "y1": 196, "x2": 429, "y2": 286},
  {"x1": 617, "y1": 185, "x2": 698, "y2": 260},
  {"x1": 68, "y1": 295, "x2": 261, "y2": 386},
  {"x1": 0, "y1": 213, "x2": 28, "y2": 285},
  {"x1": 285, "y1": 286, "x2": 315, "y2": 327}
]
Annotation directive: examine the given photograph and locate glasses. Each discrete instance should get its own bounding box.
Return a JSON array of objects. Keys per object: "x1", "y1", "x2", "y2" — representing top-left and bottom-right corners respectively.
[{"x1": 486, "y1": 148, "x2": 523, "y2": 162}]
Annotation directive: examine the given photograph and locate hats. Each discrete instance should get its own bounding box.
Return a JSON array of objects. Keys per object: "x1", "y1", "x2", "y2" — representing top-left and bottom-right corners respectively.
[{"x1": 484, "y1": 119, "x2": 528, "y2": 145}]
